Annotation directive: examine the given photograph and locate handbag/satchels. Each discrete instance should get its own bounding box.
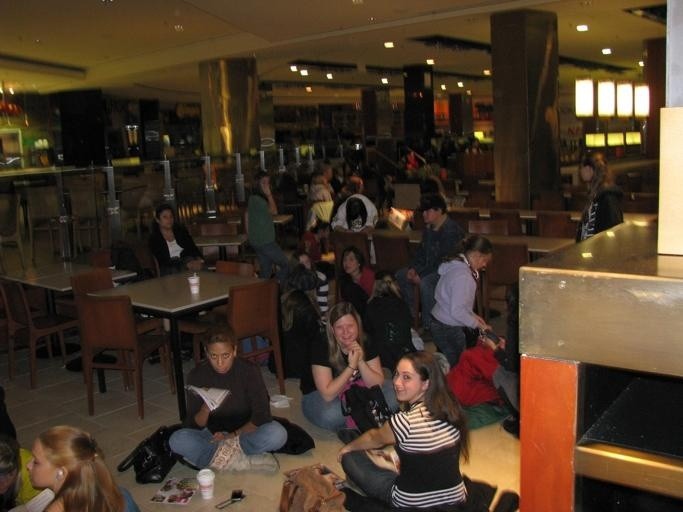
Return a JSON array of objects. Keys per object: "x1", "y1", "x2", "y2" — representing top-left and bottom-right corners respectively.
[
  {"x1": 280, "y1": 462, "x2": 350, "y2": 512},
  {"x1": 118, "y1": 427, "x2": 175, "y2": 484},
  {"x1": 339, "y1": 384, "x2": 393, "y2": 434}
]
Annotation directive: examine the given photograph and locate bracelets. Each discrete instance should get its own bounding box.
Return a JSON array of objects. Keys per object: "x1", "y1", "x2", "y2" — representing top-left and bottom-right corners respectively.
[{"x1": 347, "y1": 363, "x2": 353, "y2": 369}]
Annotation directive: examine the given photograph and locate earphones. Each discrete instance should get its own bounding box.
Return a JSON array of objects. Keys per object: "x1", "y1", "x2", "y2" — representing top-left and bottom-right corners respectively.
[{"x1": 56, "y1": 469, "x2": 64, "y2": 481}]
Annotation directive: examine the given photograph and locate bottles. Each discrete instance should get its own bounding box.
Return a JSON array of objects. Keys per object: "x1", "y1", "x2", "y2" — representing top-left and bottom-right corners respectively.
[
  {"x1": 391, "y1": 100, "x2": 399, "y2": 111},
  {"x1": 440, "y1": 112, "x2": 443, "y2": 118},
  {"x1": 353, "y1": 97, "x2": 360, "y2": 110}
]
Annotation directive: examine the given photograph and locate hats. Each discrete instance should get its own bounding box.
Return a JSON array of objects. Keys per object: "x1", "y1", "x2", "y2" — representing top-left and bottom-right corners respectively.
[{"x1": 420, "y1": 193, "x2": 445, "y2": 209}]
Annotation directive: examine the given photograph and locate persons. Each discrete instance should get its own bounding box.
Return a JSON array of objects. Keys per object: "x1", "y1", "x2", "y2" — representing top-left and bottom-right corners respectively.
[
  {"x1": 148, "y1": 203, "x2": 207, "y2": 361},
  {"x1": 575, "y1": 149, "x2": 624, "y2": 243},
  {"x1": 169, "y1": 321, "x2": 288, "y2": 473},
  {"x1": 297, "y1": 301, "x2": 384, "y2": 429},
  {"x1": 247, "y1": 135, "x2": 488, "y2": 378},
  {"x1": 430, "y1": 234, "x2": 519, "y2": 438},
  {"x1": 337, "y1": 350, "x2": 468, "y2": 510},
  {"x1": 1, "y1": 384, "x2": 141, "y2": 512}
]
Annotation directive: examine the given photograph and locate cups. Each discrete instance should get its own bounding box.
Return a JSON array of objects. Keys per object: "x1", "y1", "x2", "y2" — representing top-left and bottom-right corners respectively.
[
  {"x1": 34, "y1": 137, "x2": 48, "y2": 150},
  {"x1": 186, "y1": 276, "x2": 200, "y2": 295},
  {"x1": 615, "y1": 147, "x2": 623, "y2": 158},
  {"x1": 195, "y1": 469, "x2": 217, "y2": 500}
]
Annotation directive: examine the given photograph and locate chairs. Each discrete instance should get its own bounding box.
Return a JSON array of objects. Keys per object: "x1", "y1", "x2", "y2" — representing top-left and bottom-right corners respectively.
[
  {"x1": 1, "y1": 136, "x2": 659, "y2": 419},
  {"x1": 458, "y1": 421, "x2": 519, "y2": 512}
]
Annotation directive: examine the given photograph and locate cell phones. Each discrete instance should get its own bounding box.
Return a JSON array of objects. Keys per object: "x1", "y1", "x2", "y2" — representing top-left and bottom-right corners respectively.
[{"x1": 231, "y1": 490, "x2": 242, "y2": 501}]
